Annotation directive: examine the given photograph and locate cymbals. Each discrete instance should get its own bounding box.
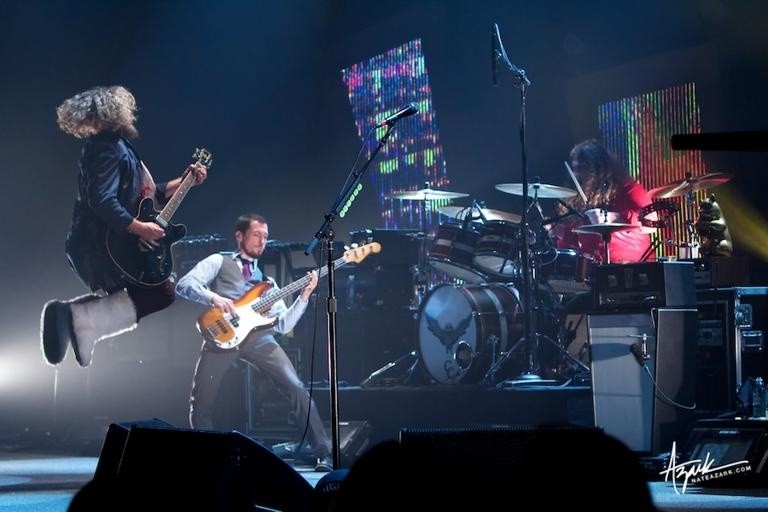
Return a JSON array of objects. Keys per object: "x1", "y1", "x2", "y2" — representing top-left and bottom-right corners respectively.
[
  {"x1": 388, "y1": 190, "x2": 469, "y2": 200},
  {"x1": 647, "y1": 177, "x2": 730, "y2": 198},
  {"x1": 437, "y1": 205, "x2": 522, "y2": 222},
  {"x1": 571, "y1": 223, "x2": 640, "y2": 233},
  {"x1": 495, "y1": 183, "x2": 578, "y2": 199}
]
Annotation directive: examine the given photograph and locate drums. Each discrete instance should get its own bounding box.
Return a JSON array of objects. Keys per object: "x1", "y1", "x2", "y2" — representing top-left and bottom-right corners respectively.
[
  {"x1": 416, "y1": 282, "x2": 527, "y2": 385},
  {"x1": 474, "y1": 220, "x2": 535, "y2": 279},
  {"x1": 428, "y1": 224, "x2": 490, "y2": 284}
]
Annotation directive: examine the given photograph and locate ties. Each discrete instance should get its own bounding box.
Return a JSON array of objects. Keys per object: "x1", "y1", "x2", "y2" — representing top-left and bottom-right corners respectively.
[{"x1": 238, "y1": 256, "x2": 252, "y2": 283}]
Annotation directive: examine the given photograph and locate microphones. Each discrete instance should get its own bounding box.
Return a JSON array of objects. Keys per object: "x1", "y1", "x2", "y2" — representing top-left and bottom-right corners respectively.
[
  {"x1": 629, "y1": 341, "x2": 648, "y2": 369},
  {"x1": 374, "y1": 102, "x2": 420, "y2": 129},
  {"x1": 406, "y1": 264, "x2": 427, "y2": 285},
  {"x1": 491, "y1": 19, "x2": 500, "y2": 87},
  {"x1": 453, "y1": 340, "x2": 475, "y2": 373}
]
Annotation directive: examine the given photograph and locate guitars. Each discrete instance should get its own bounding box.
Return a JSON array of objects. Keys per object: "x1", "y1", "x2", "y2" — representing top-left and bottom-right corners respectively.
[
  {"x1": 535, "y1": 248, "x2": 596, "y2": 294},
  {"x1": 196, "y1": 237, "x2": 380, "y2": 352},
  {"x1": 106, "y1": 147, "x2": 213, "y2": 286}
]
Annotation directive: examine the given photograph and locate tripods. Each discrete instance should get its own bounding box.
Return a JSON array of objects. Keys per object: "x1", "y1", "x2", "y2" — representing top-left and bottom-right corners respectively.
[
  {"x1": 359, "y1": 288, "x2": 437, "y2": 389},
  {"x1": 476, "y1": 40, "x2": 591, "y2": 389}
]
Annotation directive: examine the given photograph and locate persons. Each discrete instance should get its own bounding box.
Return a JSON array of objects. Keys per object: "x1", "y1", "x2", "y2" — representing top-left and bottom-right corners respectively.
[
  {"x1": 545, "y1": 139, "x2": 660, "y2": 264},
  {"x1": 39, "y1": 85, "x2": 208, "y2": 368},
  {"x1": 174, "y1": 213, "x2": 333, "y2": 471}
]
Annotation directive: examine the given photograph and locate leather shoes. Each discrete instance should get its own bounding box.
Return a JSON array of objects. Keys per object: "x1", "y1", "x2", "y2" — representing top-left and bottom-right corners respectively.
[{"x1": 314, "y1": 455, "x2": 334, "y2": 472}]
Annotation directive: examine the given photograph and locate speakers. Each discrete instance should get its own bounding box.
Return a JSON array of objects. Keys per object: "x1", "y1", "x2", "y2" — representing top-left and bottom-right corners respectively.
[
  {"x1": 301, "y1": 418, "x2": 372, "y2": 469},
  {"x1": 343, "y1": 228, "x2": 423, "y2": 312},
  {"x1": 398, "y1": 425, "x2": 605, "y2": 488},
  {"x1": 339, "y1": 308, "x2": 418, "y2": 385},
  {"x1": 587, "y1": 308, "x2": 697, "y2": 453},
  {"x1": 664, "y1": 420, "x2": 768, "y2": 488}
]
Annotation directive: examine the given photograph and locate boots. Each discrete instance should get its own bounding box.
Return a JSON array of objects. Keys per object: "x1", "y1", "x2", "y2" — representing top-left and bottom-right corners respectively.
[
  {"x1": 68, "y1": 286, "x2": 140, "y2": 367},
  {"x1": 41, "y1": 292, "x2": 103, "y2": 365}
]
